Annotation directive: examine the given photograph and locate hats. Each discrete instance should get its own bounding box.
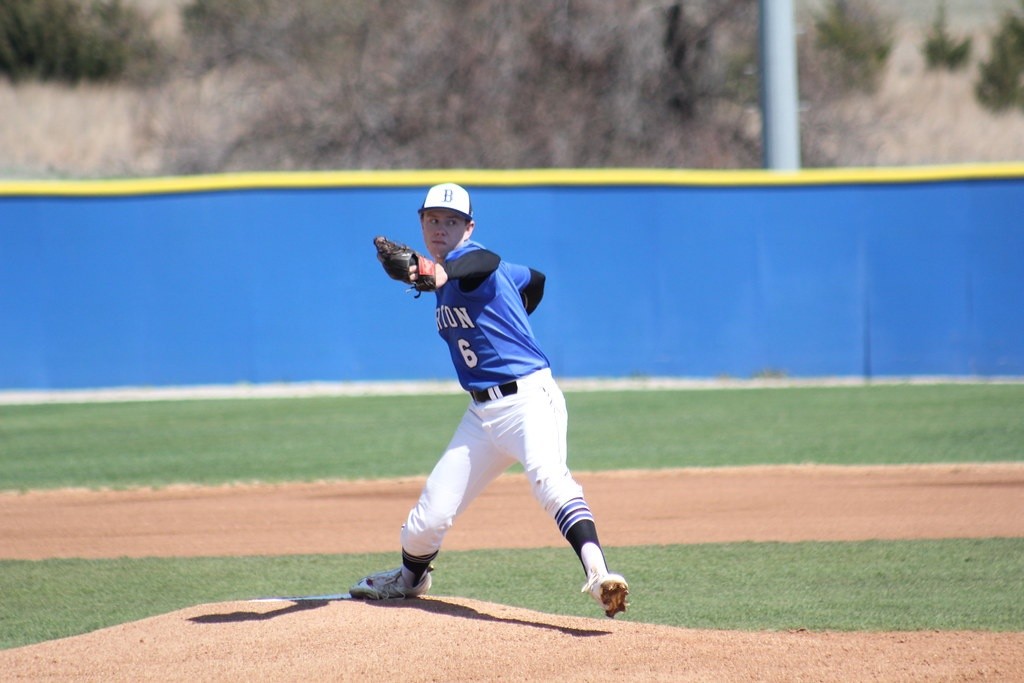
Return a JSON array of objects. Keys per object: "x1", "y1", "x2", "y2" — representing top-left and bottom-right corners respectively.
[{"x1": 418, "y1": 183, "x2": 472, "y2": 221}]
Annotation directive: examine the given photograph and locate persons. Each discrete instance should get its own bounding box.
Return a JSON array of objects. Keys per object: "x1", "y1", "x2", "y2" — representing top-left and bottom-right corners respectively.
[{"x1": 349, "y1": 180, "x2": 629, "y2": 620}]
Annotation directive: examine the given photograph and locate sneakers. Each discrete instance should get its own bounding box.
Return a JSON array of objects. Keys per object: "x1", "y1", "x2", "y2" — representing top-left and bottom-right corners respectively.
[
  {"x1": 590, "y1": 574, "x2": 629, "y2": 619},
  {"x1": 348, "y1": 567, "x2": 433, "y2": 600}
]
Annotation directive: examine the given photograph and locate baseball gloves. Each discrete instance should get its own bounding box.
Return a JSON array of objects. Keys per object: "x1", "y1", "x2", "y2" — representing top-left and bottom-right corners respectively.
[{"x1": 374, "y1": 234, "x2": 439, "y2": 300}]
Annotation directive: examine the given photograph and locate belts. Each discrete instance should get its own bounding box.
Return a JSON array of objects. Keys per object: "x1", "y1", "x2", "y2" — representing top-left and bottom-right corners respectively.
[{"x1": 469, "y1": 382, "x2": 517, "y2": 402}]
